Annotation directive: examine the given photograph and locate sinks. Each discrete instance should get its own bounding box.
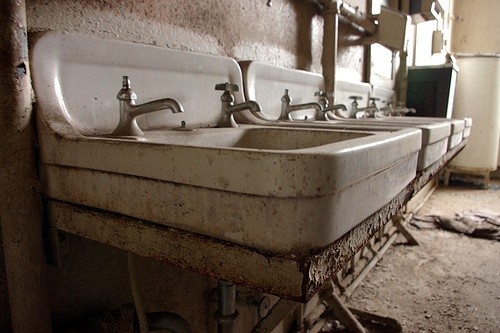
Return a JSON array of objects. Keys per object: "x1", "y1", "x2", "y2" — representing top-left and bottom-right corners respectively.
[
  {"x1": 377, "y1": 117, "x2": 466, "y2": 150},
  {"x1": 330, "y1": 116, "x2": 452, "y2": 173},
  {"x1": 463, "y1": 117, "x2": 472, "y2": 138},
  {"x1": 87, "y1": 125, "x2": 423, "y2": 255}
]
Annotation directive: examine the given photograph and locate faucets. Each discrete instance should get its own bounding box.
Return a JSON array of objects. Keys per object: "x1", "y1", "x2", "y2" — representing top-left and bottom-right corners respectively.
[
  {"x1": 394, "y1": 101, "x2": 416, "y2": 116},
  {"x1": 214, "y1": 82, "x2": 260, "y2": 129},
  {"x1": 369, "y1": 97, "x2": 391, "y2": 118},
  {"x1": 276, "y1": 89, "x2": 324, "y2": 121},
  {"x1": 115, "y1": 74, "x2": 184, "y2": 136},
  {"x1": 348, "y1": 95, "x2": 379, "y2": 119},
  {"x1": 382, "y1": 100, "x2": 409, "y2": 117},
  {"x1": 314, "y1": 90, "x2": 347, "y2": 121}
]
